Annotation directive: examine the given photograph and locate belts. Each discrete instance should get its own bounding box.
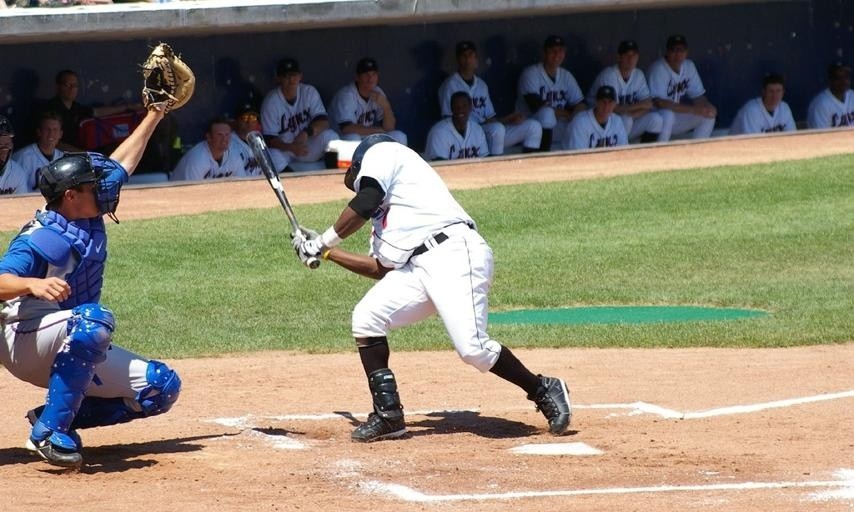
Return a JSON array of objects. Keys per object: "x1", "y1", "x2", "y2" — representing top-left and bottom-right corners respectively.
[{"x1": 405, "y1": 223, "x2": 475, "y2": 265}]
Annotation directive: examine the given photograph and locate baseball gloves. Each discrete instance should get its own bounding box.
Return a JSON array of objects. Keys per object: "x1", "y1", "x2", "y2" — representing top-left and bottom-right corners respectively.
[{"x1": 140, "y1": 42, "x2": 196, "y2": 117}]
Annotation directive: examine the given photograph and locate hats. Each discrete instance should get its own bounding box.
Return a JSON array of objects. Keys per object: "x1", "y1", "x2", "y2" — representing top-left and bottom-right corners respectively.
[
  {"x1": 596, "y1": 85, "x2": 617, "y2": 102},
  {"x1": 827, "y1": 60, "x2": 852, "y2": 76},
  {"x1": 456, "y1": 41, "x2": 477, "y2": 55},
  {"x1": 237, "y1": 101, "x2": 259, "y2": 116},
  {"x1": 618, "y1": 39, "x2": 639, "y2": 55},
  {"x1": 357, "y1": 57, "x2": 378, "y2": 74},
  {"x1": 667, "y1": 34, "x2": 688, "y2": 50},
  {"x1": 277, "y1": 58, "x2": 301, "y2": 77},
  {"x1": 546, "y1": 35, "x2": 566, "y2": 48}
]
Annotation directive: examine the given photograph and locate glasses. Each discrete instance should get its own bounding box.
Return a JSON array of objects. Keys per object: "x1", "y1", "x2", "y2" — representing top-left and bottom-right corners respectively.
[{"x1": 239, "y1": 114, "x2": 259, "y2": 122}]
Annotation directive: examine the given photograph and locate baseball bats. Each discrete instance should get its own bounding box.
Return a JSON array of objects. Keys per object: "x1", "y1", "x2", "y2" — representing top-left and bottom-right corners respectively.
[{"x1": 245, "y1": 129, "x2": 321, "y2": 270}]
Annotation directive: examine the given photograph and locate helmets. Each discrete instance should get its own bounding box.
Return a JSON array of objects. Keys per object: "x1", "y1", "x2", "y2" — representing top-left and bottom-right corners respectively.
[
  {"x1": 40, "y1": 150, "x2": 106, "y2": 204},
  {"x1": 344, "y1": 134, "x2": 397, "y2": 192},
  {"x1": 0, "y1": 114, "x2": 16, "y2": 138}
]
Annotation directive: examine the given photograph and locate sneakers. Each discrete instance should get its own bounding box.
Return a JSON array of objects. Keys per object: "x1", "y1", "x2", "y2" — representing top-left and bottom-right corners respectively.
[
  {"x1": 25, "y1": 403, "x2": 46, "y2": 430},
  {"x1": 25, "y1": 433, "x2": 83, "y2": 468},
  {"x1": 351, "y1": 408, "x2": 407, "y2": 443},
  {"x1": 527, "y1": 374, "x2": 572, "y2": 435}
]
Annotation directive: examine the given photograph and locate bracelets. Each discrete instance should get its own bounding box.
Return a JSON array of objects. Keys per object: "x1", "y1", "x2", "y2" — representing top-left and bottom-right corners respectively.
[{"x1": 322, "y1": 245, "x2": 336, "y2": 260}]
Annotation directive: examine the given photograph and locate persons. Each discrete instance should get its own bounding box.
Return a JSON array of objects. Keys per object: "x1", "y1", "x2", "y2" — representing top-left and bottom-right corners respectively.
[
  {"x1": 287, "y1": 130, "x2": 575, "y2": 445},
  {"x1": 0, "y1": 116, "x2": 28, "y2": 194},
  {"x1": 12, "y1": 116, "x2": 66, "y2": 195},
  {"x1": 729, "y1": 78, "x2": 799, "y2": 135},
  {"x1": 0, "y1": 40, "x2": 200, "y2": 469},
  {"x1": 806, "y1": 60, "x2": 852, "y2": 131},
  {"x1": 45, "y1": 69, "x2": 139, "y2": 144}
]
